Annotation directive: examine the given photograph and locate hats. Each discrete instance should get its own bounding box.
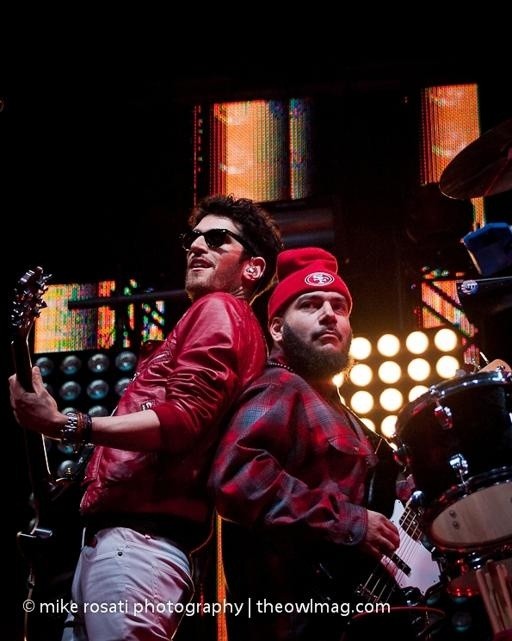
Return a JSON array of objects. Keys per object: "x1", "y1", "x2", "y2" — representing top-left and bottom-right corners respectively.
[{"x1": 267, "y1": 247, "x2": 353, "y2": 321}]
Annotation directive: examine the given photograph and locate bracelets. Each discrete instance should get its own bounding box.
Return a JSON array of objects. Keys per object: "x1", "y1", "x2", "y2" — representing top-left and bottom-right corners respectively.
[{"x1": 61, "y1": 413, "x2": 92, "y2": 447}]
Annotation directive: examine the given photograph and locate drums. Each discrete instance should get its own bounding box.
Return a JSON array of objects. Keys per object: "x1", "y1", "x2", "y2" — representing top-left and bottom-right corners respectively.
[
  {"x1": 395, "y1": 364, "x2": 512, "y2": 552},
  {"x1": 442, "y1": 543, "x2": 512, "y2": 598}
]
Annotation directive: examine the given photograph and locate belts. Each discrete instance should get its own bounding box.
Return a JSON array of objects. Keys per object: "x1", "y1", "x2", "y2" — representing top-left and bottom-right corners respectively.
[{"x1": 82, "y1": 517, "x2": 195, "y2": 551}]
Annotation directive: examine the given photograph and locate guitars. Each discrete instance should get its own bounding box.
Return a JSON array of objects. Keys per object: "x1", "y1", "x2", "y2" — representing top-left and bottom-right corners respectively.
[
  {"x1": 306, "y1": 362, "x2": 508, "y2": 641},
  {"x1": 11, "y1": 266, "x2": 96, "y2": 578}
]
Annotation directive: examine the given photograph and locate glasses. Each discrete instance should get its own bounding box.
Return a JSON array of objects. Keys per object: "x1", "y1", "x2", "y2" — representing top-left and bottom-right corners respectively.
[{"x1": 178, "y1": 228, "x2": 255, "y2": 256}]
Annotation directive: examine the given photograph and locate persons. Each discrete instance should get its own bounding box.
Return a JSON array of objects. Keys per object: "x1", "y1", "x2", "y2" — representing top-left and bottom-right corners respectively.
[
  {"x1": 8, "y1": 194, "x2": 284, "y2": 641},
  {"x1": 207, "y1": 246, "x2": 400, "y2": 641}
]
